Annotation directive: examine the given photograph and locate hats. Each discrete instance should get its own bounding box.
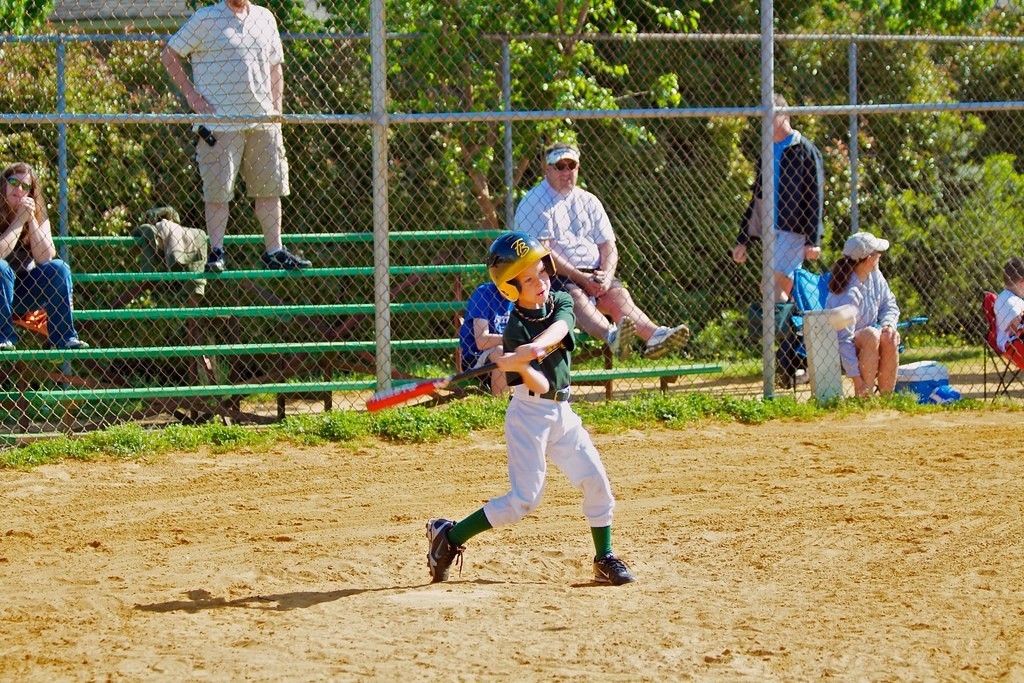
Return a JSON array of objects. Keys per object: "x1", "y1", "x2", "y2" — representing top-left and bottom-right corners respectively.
[
  {"x1": 545, "y1": 149, "x2": 579, "y2": 164},
  {"x1": 842, "y1": 232, "x2": 889, "y2": 260}
]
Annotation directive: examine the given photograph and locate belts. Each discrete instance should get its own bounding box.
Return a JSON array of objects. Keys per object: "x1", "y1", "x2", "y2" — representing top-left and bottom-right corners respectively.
[{"x1": 529, "y1": 388, "x2": 569, "y2": 402}]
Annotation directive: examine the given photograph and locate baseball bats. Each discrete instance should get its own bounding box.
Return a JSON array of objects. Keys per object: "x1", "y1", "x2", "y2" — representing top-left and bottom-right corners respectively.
[{"x1": 364, "y1": 346, "x2": 546, "y2": 412}]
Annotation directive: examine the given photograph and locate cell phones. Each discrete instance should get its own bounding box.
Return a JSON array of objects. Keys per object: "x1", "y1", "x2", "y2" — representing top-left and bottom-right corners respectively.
[{"x1": 197, "y1": 125, "x2": 216, "y2": 147}]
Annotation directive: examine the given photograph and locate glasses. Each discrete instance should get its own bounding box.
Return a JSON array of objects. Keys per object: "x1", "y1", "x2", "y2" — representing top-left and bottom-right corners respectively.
[
  {"x1": 549, "y1": 162, "x2": 577, "y2": 171},
  {"x1": 8, "y1": 178, "x2": 33, "y2": 190}
]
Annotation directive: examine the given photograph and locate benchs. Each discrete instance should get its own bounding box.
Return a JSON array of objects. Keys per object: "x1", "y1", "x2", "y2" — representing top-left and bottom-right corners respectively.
[{"x1": 0, "y1": 229, "x2": 722, "y2": 436}]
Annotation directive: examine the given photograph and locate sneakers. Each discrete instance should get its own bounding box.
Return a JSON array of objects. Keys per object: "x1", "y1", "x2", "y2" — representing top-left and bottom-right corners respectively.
[
  {"x1": 608, "y1": 316, "x2": 637, "y2": 363},
  {"x1": 0, "y1": 340, "x2": 16, "y2": 350},
  {"x1": 593, "y1": 553, "x2": 637, "y2": 586},
  {"x1": 205, "y1": 248, "x2": 226, "y2": 271},
  {"x1": 50, "y1": 337, "x2": 89, "y2": 349},
  {"x1": 425, "y1": 518, "x2": 466, "y2": 581},
  {"x1": 644, "y1": 325, "x2": 689, "y2": 360},
  {"x1": 262, "y1": 249, "x2": 312, "y2": 269}
]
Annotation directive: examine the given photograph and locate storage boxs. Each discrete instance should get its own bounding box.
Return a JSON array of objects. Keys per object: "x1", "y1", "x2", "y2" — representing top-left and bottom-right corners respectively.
[{"x1": 894, "y1": 360, "x2": 949, "y2": 404}]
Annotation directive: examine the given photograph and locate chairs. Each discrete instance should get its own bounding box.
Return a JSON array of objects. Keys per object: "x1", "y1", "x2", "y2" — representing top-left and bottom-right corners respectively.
[
  {"x1": 781, "y1": 268, "x2": 928, "y2": 399},
  {"x1": 981, "y1": 290, "x2": 1024, "y2": 406}
]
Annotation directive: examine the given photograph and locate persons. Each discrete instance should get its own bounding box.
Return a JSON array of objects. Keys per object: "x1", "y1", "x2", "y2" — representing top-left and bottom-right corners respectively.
[
  {"x1": 423, "y1": 232, "x2": 634, "y2": 588},
  {"x1": 731, "y1": 93, "x2": 822, "y2": 386},
  {"x1": 994, "y1": 257, "x2": 1024, "y2": 353},
  {"x1": 0, "y1": 162, "x2": 89, "y2": 350},
  {"x1": 513, "y1": 144, "x2": 690, "y2": 361},
  {"x1": 162, "y1": 0, "x2": 313, "y2": 272},
  {"x1": 825, "y1": 233, "x2": 901, "y2": 402},
  {"x1": 459, "y1": 282, "x2": 516, "y2": 399}
]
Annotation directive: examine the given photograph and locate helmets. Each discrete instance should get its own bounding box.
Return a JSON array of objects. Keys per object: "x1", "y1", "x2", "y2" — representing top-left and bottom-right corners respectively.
[{"x1": 487, "y1": 232, "x2": 556, "y2": 303}]
